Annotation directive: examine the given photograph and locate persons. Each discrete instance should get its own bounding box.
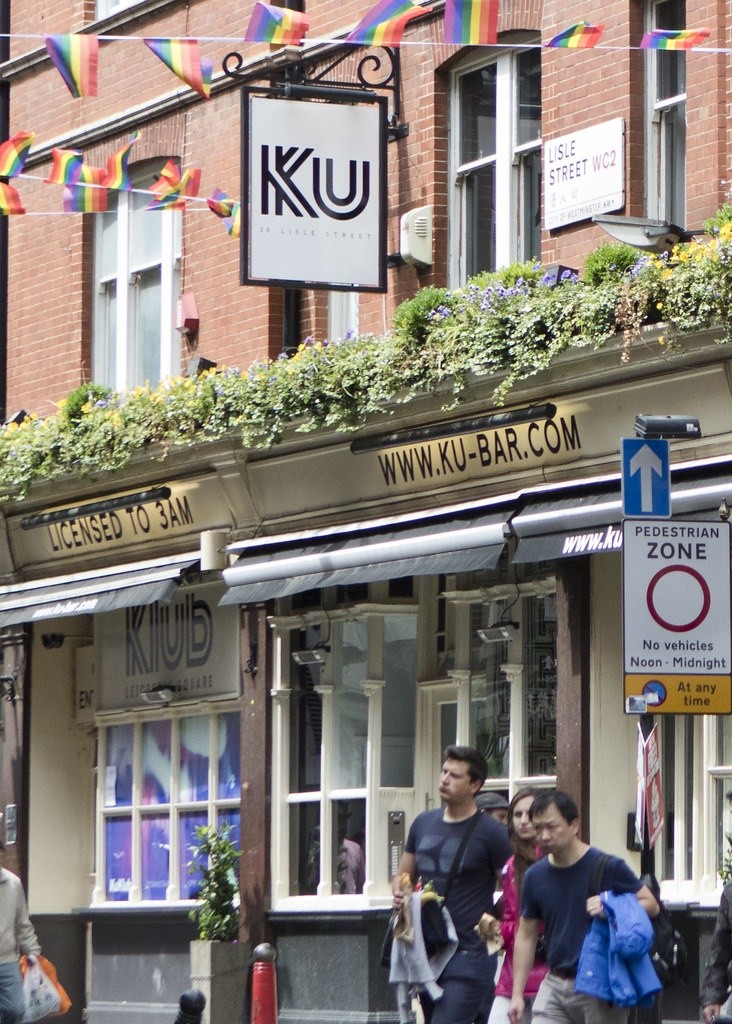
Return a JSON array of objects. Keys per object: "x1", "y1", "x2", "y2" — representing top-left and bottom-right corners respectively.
[
  {"x1": 305, "y1": 808, "x2": 365, "y2": 894},
  {"x1": 700, "y1": 789, "x2": 732, "y2": 1024},
  {"x1": 392, "y1": 746, "x2": 513, "y2": 1024},
  {"x1": 508, "y1": 791, "x2": 659, "y2": 1023},
  {"x1": 0, "y1": 840, "x2": 42, "y2": 1023},
  {"x1": 474, "y1": 786, "x2": 549, "y2": 1024}
]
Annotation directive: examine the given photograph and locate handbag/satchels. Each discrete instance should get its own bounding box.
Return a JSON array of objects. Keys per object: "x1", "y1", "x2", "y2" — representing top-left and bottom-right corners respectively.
[
  {"x1": 19, "y1": 954, "x2": 71, "y2": 1024},
  {"x1": 380, "y1": 899, "x2": 449, "y2": 968}
]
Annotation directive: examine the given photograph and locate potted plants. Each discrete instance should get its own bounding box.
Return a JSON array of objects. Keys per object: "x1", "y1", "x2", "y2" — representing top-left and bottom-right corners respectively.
[{"x1": 185, "y1": 820, "x2": 249, "y2": 1024}]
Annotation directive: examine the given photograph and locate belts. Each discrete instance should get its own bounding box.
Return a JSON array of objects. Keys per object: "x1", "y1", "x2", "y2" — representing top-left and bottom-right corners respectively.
[{"x1": 550, "y1": 967, "x2": 578, "y2": 979}]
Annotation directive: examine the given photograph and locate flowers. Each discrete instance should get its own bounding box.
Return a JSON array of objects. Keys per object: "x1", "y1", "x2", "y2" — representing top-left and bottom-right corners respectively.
[{"x1": 0, "y1": 202, "x2": 732, "y2": 505}]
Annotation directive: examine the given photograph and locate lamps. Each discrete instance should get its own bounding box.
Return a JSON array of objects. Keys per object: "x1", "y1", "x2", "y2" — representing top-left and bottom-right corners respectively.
[
  {"x1": 477, "y1": 620, "x2": 521, "y2": 644},
  {"x1": 140, "y1": 684, "x2": 176, "y2": 705},
  {"x1": 590, "y1": 212, "x2": 712, "y2": 256},
  {"x1": 185, "y1": 356, "x2": 219, "y2": 379},
  {"x1": 635, "y1": 411, "x2": 701, "y2": 443},
  {"x1": 291, "y1": 647, "x2": 325, "y2": 666},
  {"x1": 199, "y1": 529, "x2": 229, "y2": 571},
  {"x1": 4, "y1": 409, "x2": 33, "y2": 426}
]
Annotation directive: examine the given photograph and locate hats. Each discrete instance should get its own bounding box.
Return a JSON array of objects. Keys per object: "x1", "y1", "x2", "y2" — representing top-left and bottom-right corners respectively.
[{"x1": 475, "y1": 792, "x2": 511, "y2": 812}]
